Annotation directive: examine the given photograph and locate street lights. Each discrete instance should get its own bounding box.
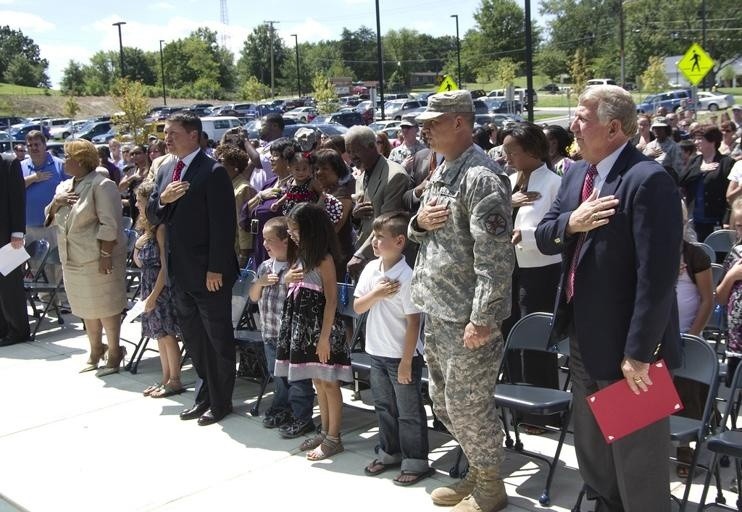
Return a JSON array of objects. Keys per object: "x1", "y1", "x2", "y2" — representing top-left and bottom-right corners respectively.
[
  {"x1": 290, "y1": 34, "x2": 301, "y2": 98},
  {"x1": 159, "y1": 40, "x2": 168, "y2": 107},
  {"x1": 113, "y1": 21, "x2": 127, "y2": 82},
  {"x1": 450, "y1": 14, "x2": 463, "y2": 89}
]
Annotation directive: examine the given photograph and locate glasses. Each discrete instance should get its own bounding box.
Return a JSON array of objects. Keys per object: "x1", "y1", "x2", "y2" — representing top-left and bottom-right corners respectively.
[
  {"x1": 26, "y1": 142, "x2": 43, "y2": 148},
  {"x1": 226, "y1": 140, "x2": 233, "y2": 143},
  {"x1": 376, "y1": 141, "x2": 383, "y2": 144},
  {"x1": 149, "y1": 148, "x2": 160, "y2": 153},
  {"x1": 402, "y1": 126, "x2": 414, "y2": 129},
  {"x1": 670, "y1": 119, "x2": 675, "y2": 121},
  {"x1": 286, "y1": 230, "x2": 299, "y2": 235},
  {"x1": 130, "y1": 151, "x2": 144, "y2": 156},
  {"x1": 17, "y1": 149, "x2": 26, "y2": 152},
  {"x1": 680, "y1": 125, "x2": 689, "y2": 127},
  {"x1": 638, "y1": 124, "x2": 650, "y2": 127},
  {"x1": 720, "y1": 129, "x2": 734, "y2": 133},
  {"x1": 218, "y1": 159, "x2": 227, "y2": 162},
  {"x1": 320, "y1": 137, "x2": 327, "y2": 139},
  {"x1": 64, "y1": 154, "x2": 71, "y2": 160},
  {"x1": 122, "y1": 150, "x2": 129, "y2": 153}
]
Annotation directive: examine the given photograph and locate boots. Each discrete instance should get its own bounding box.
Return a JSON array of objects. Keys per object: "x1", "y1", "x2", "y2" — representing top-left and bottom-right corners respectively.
[
  {"x1": 451, "y1": 476, "x2": 507, "y2": 512},
  {"x1": 430, "y1": 473, "x2": 479, "y2": 504}
]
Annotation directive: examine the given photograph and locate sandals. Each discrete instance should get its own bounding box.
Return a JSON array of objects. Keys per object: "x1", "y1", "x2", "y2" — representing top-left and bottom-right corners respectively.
[
  {"x1": 678, "y1": 448, "x2": 693, "y2": 476},
  {"x1": 299, "y1": 434, "x2": 326, "y2": 451},
  {"x1": 306, "y1": 434, "x2": 344, "y2": 460}
]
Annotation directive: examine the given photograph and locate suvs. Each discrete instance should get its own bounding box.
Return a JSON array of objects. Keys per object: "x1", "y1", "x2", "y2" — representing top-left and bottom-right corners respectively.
[
  {"x1": 637, "y1": 90, "x2": 695, "y2": 119},
  {"x1": 373, "y1": 97, "x2": 421, "y2": 120},
  {"x1": 476, "y1": 87, "x2": 540, "y2": 112},
  {"x1": 229, "y1": 104, "x2": 257, "y2": 117},
  {"x1": 158, "y1": 107, "x2": 181, "y2": 120}
]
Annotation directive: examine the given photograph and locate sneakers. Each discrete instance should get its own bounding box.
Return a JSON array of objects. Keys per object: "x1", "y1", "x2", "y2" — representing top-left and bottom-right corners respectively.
[
  {"x1": 35, "y1": 306, "x2": 52, "y2": 314},
  {"x1": 263, "y1": 409, "x2": 289, "y2": 429},
  {"x1": 279, "y1": 419, "x2": 315, "y2": 438},
  {"x1": 36, "y1": 303, "x2": 54, "y2": 314},
  {"x1": 61, "y1": 303, "x2": 72, "y2": 314}
]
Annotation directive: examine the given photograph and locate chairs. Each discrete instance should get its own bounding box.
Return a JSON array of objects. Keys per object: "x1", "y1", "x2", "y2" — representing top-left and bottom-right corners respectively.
[
  {"x1": 251, "y1": 284, "x2": 366, "y2": 430},
  {"x1": 416, "y1": 363, "x2": 514, "y2": 480},
  {"x1": 699, "y1": 359, "x2": 737, "y2": 511},
  {"x1": 116, "y1": 213, "x2": 135, "y2": 231},
  {"x1": 178, "y1": 270, "x2": 274, "y2": 375},
  {"x1": 14, "y1": 239, "x2": 52, "y2": 284},
  {"x1": 699, "y1": 227, "x2": 737, "y2": 257},
  {"x1": 199, "y1": 282, "x2": 269, "y2": 371},
  {"x1": 572, "y1": 334, "x2": 720, "y2": 512},
  {"x1": 99, "y1": 274, "x2": 150, "y2": 369},
  {"x1": 22, "y1": 243, "x2": 87, "y2": 339},
  {"x1": 130, "y1": 316, "x2": 186, "y2": 376},
  {"x1": 348, "y1": 308, "x2": 437, "y2": 430},
  {"x1": 467, "y1": 312, "x2": 571, "y2": 505},
  {"x1": 116, "y1": 230, "x2": 155, "y2": 308},
  {"x1": 701, "y1": 261, "x2": 733, "y2": 395}
]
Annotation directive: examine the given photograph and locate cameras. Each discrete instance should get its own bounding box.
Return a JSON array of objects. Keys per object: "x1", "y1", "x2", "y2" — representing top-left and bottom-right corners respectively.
[{"x1": 250, "y1": 218, "x2": 260, "y2": 235}]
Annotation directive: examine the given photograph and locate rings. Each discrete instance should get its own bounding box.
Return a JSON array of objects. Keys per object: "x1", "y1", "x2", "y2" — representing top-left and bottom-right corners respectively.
[
  {"x1": 593, "y1": 214, "x2": 598, "y2": 220},
  {"x1": 634, "y1": 379, "x2": 641, "y2": 384},
  {"x1": 69, "y1": 200, "x2": 71, "y2": 204}
]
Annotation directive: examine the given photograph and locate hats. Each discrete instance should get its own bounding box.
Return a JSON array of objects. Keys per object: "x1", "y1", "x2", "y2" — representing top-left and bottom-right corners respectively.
[
  {"x1": 148, "y1": 135, "x2": 157, "y2": 140},
  {"x1": 293, "y1": 128, "x2": 316, "y2": 143},
  {"x1": 652, "y1": 117, "x2": 670, "y2": 127},
  {"x1": 414, "y1": 90, "x2": 475, "y2": 122},
  {"x1": 400, "y1": 115, "x2": 420, "y2": 128},
  {"x1": 731, "y1": 104, "x2": 742, "y2": 111}
]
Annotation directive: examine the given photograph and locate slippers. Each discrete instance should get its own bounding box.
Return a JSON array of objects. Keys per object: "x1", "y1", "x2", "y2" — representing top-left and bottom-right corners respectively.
[
  {"x1": 364, "y1": 459, "x2": 401, "y2": 476},
  {"x1": 143, "y1": 382, "x2": 166, "y2": 396},
  {"x1": 150, "y1": 384, "x2": 186, "y2": 398},
  {"x1": 392, "y1": 465, "x2": 436, "y2": 486}
]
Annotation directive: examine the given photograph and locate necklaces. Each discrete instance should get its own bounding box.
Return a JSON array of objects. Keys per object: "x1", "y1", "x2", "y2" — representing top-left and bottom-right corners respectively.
[
  {"x1": 75, "y1": 175, "x2": 85, "y2": 182},
  {"x1": 277, "y1": 175, "x2": 293, "y2": 188}
]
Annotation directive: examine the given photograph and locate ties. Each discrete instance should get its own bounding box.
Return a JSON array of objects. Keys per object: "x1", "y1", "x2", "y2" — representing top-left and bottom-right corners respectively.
[
  {"x1": 363, "y1": 170, "x2": 370, "y2": 189},
  {"x1": 564, "y1": 165, "x2": 597, "y2": 302},
  {"x1": 172, "y1": 160, "x2": 185, "y2": 182},
  {"x1": 429, "y1": 153, "x2": 437, "y2": 170}
]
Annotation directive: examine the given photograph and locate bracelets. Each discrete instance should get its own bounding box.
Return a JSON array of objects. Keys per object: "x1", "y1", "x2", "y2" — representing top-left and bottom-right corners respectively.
[
  {"x1": 134, "y1": 247, "x2": 142, "y2": 251},
  {"x1": 100, "y1": 249, "x2": 112, "y2": 259},
  {"x1": 257, "y1": 191, "x2": 263, "y2": 204}
]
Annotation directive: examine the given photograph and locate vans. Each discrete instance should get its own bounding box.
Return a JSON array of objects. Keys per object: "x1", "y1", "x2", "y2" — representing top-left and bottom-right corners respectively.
[
  {"x1": 313, "y1": 111, "x2": 366, "y2": 129},
  {"x1": 582, "y1": 78, "x2": 617, "y2": 87},
  {"x1": 197, "y1": 117, "x2": 241, "y2": 142},
  {"x1": 63, "y1": 123, "x2": 110, "y2": 143},
  {"x1": 42, "y1": 116, "x2": 73, "y2": 134}
]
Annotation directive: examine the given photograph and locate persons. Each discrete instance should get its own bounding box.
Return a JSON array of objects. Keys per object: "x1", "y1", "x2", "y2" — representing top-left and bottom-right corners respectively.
[
  {"x1": 310, "y1": 149, "x2": 356, "y2": 282},
  {"x1": 501, "y1": 122, "x2": 564, "y2": 435},
  {"x1": 684, "y1": 111, "x2": 694, "y2": 120},
  {"x1": 677, "y1": 100, "x2": 688, "y2": 119},
  {"x1": 408, "y1": 89, "x2": 514, "y2": 512},
  {"x1": 404, "y1": 146, "x2": 444, "y2": 213},
  {"x1": 728, "y1": 133, "x2": 742, "y2": 206},
  {"x1": 201, "y1": 132, "x2": 208, "y2": 158},
  {"x1": 292, "y1": 128, "x2": 319, "y2": 156},
  {"x1": 109, "y1": 139, "x2": 123, "y2": 171},
  {"x1": 486, "y1": 124, "x2": 505, "y2": 153},
  {"x1": 721, "y1": 112, "x2": 730, "y2": 123},
  {"x1": 214, "y1": 145, "x2": 258, "y2": 269},
  {"x1": 0, "y1": 154, "x2": 30, "y2": 348},
  {"x1": 642, "y1": 117, "x2": 677, "y2": 167},
  {"x1": 535, "y1": 84, "x2": 684, "y2": 512},
  {"x1": 488, "y1": 146, "x2": 518, "y2": 179},
  {"x1": 252, "y1": 141, "x2": 258, "y2": 148},
  {"x1": 654, "y1": 107, "x2": 668, "y2": 119},
  {"x1": 676, "y1": 197, "x2": 715, "y2": 340},
  {"x1": 344, "y1": 126, "x2": 411, "y2": 401},
  {"x1": 19, "y1": 130, "x2": 68, "y2": 312},
  {"x1": 714, "y1": 196, "x2": 742, "y2": 389},
  {"x1": 709, "y1": 115, "x2": 721, "y2": 129},
  {"x1": 665, "y1": 114, "x2": 680, "y2": 144},
  {"x1": 239, "y1": 138, "x2": 300, "y2": 313},
  {"x1": 631, "y1": 114, "x2": 656, "y2": 154},
  {"x1": 146, "y1": 112, "x2": 243, "y2": 426},
  {"x1": 353, "y1": 212, "x2": 434, "y2": 486},
  {"x1": 119, "y1": 145, "x2": 136, "y2": 177},
  {"x1": 319, "y1": 133, "x2": 330, "y2": 143},
  {"x1": 148, "y1": 138, "x2": 165, "y2": 169},
  {"x1": 118, "y1": 146, "x2": 147, "y2": 228},
  {"x1": 673, "y1": 139, "x2": 697, "y2": 187},
  {"x1": 98, "y1": 146, "x2": 119, "y2": 186},
  {"x1": 271, "y1": 145, "x2": 322, "y2": 217},
  {"x1": 275, "y1": 203, "x2": 340, "y2": 461},
  {"x1": 43, "y1": 137, "x2": 128, "y2": 372},
  {"x1": 679, "y1": 118, "x2": 693, "y2": 140},
  {"x1": 542, "y1": 126, "x2": 575, "y2": 178},
  {"x1": 322, "y1": 134, "x2": 355, "y2": 168},
  {"x1": 730, "y1": 104, "x2": 742, "y2": 144},
  {"x1": 678, "y1": 127, "x2": 736, "y2": 265},
  {"x1": 688, "y1": 122, "x2": 701, "y2": 139},
  {"x1": 718, "y1": 122, "x2": 742, "y2": 161},
  {"x1": 133, "y1": 180, "x2": 169, "y2": 396},
  {"x1": 14, "y1": 144, "x2": 26, "y2": 161},
  {"x1": 213, "y1": 113, "x2": 285, "y2": 195},
  {"x1": 470, "y1": 124, "x2": 495, "y2": 155},
  {"x1": 374, "y1": 131, "x2": 393, "y2": 158},
  {"x1": 387, "y1": 117, "x2": 428, "y2": 174},
  {"x1": 249, "y1": 217, "x2": 317, "y2": 438}
]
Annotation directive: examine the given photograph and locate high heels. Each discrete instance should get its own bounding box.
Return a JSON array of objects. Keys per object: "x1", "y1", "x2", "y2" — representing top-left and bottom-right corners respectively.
[
  {"x1": 96, "y1": 346, "x2": 126, "y2": 377},
  {"x1": 80, "y1": 344, "x2": 108, "y2": 374}
]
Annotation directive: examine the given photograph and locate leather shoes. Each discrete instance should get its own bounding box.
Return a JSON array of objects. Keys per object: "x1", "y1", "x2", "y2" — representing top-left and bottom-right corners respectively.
[
  {"x1": 0, "y1": 334, "x2": 30, "y2": 346},
  {"x1": 180, "y1": 400, "x2": 210, "y2": 421},
  {"x1": 197, "y1": 407, "x2": 232, "y2": 426}
]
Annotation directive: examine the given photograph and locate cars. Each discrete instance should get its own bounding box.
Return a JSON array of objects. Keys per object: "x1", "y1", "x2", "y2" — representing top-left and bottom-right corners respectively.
[
  {"x1": 53, "y1": 121, "x2": 91, "y2": 137},
  {"x1": 5, "y1": 123, "x2": 50, "y2": 144},
  {"x1": 697, "y1": 90, "x2": 738, "y2": 112},
  {"x1": 489, "y1": 98, "x2": 524, "y2": 115},
  {"x1": 245, "y1": 120, "x2": 265, "y2": 139},
  {"x1": 208, "y1": 108, "x2": 244, "y2": 117},
  {"x1": 282, "y1": 124, "x2": 347, "y2": 139},
  {"x1": 366, "y1": 117, "x2": 403, "y2": 139},
  {"x1": 283, "y1": 104, "x2": 313, "y2": 122},
  {"x1": 92, "y1": 125, "x2": 131, "y2": 146}
]
